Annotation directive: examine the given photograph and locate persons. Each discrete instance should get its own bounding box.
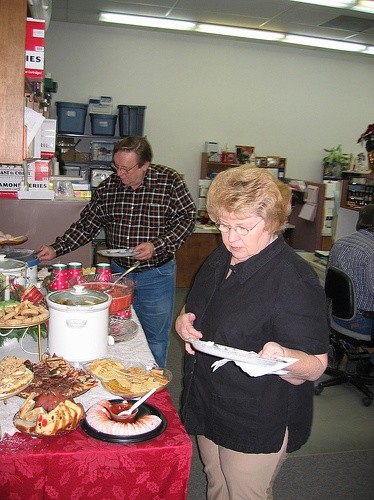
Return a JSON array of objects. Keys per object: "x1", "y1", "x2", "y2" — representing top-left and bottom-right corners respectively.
[
  {"x1": 175, "y1": 162, "x2": 329, "y2": 500},
  {"x1": 326, "y1": 205, "x2": 374, "y2": 370},
  {"x1": 34, "y1": 135, "x2": 197, "y2": 368}
]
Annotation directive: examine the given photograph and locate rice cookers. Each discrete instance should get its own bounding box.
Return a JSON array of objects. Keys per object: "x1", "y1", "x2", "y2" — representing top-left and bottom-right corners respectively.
[
  {"x1": 5, "y1": 253, "x2": 39, "y2": 288},
  {"x1": 45, "y1": 285, "x2": 114, "y2": 369},
  {"x1": 0, "y1": 254, "x2": 28, "y2": 302}
]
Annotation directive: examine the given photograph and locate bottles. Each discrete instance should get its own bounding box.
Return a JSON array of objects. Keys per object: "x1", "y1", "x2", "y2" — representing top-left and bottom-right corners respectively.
[
  {"x1": 56, "y1": 144, "x2": 76, "y2": 161},
  {"x1": 207, "y1": 142, "x2": 218, "y2": 157}
]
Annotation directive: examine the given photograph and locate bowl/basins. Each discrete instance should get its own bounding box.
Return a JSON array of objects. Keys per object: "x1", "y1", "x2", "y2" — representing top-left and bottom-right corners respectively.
[
  {"x1": 101, "y1": 368, "x2": 174, "y2": 398},
  {"x1": 69, "y1": 274, "x2": 135, "y2": 315},
  {"x1": 108, "y1": 402, "x2": 139, "y2": 425}
]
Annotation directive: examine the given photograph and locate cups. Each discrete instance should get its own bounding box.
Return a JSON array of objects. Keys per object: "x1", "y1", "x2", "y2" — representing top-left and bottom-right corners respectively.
[
  {"x1": 95, "y1": 263, "x2": 112, "y2": 288},
  {"x1": 67, "y1": 262, "x2": 83, "y2": 284},
  {"x1": 348, "y1": 183, "x2": 373, "y2": 206},
  {"x1": 48, "y1": 264, "x2": 68, "y2": 294}
]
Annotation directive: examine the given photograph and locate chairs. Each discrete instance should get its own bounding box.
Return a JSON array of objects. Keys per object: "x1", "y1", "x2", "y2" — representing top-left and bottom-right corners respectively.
[{"x1": 314, "y1": 266, "x2": 374, "y2": 406}]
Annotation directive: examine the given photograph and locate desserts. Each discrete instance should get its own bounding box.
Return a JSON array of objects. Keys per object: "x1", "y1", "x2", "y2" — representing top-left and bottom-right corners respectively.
[{"x1": 85, "y1": 399, "x2": 162, "y2": 436}]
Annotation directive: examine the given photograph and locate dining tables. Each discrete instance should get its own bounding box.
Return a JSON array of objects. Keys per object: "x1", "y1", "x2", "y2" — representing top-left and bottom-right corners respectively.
[{"x1": 0, "y1": 305, "x2": 193, "y2": 500}]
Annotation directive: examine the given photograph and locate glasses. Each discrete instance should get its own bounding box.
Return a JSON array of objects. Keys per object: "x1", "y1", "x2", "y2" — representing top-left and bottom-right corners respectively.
[
  {"x1": 215, "y1": 219, "x2": 264, "y2": 236},
  {"x1": 113, "y1": 161, "x2": 144, "y2": 174}
]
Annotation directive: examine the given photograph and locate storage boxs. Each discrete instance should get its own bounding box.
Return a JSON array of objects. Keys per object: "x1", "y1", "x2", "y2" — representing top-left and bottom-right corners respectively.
[
  {"x1": 23, "y1": 17, "x2": 47, "y2": 83},
  {"x1": 2, "y1": 160, "x2": 93, "y2": 201},
  {"x1": 55, "y1": 96, "x2": 118, "y2": 137},
  {"x1": 88, "y1": 140, "x2": 116, "y2": 164}
]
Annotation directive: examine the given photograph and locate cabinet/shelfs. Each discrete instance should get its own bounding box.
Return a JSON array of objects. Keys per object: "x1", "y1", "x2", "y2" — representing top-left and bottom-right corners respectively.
[
  {"x1": 199, "y1": 153, "x2": 281, "y2": 215},
  {"x1": 57, "y1": 133, "x2": 123, "y2": 166},
  {"x1": 0, "y1": 0, "x2": 50, "y2": 164}
]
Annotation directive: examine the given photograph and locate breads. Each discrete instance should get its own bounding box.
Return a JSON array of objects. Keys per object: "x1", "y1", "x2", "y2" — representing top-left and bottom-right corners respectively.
[
  {"x1": 13, "y1": 392, "x2": 83, "y2": 436},
  {"x1": 0, "y1": 355, "x2": 98, "y2": 400},
  {"x1": 0, "y1": 302, "x2": 50, "y2": 326}
]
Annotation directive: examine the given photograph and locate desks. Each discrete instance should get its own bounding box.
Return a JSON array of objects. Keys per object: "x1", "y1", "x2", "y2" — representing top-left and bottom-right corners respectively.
[{"x1": 176, "y1": 216, "x2": 223, "y2": 287}]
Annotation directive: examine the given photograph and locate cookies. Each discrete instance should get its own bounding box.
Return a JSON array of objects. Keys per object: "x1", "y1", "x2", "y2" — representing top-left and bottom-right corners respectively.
[{"x1": 87, "y1": 358, "x2": 169, "y2": 395}]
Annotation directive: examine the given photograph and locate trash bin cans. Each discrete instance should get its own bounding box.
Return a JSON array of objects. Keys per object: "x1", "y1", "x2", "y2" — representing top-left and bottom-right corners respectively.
[{"x1": 93, "y1": 238, "x2": 111, "y2": 266}]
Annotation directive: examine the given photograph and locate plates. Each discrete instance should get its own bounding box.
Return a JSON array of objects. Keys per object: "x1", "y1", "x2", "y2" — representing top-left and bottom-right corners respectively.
[
  {"x1": 108, "y1": 315, "x2": 138, "y2": 342},
  {"x1": 72, "y1": 389, "x2": 90, "y2": 399},
  {"x1": 0, "y1": 248, "x2": 34, "y2": 259},
  {"x1": 80, "y1": 399, "x2": 167, "y2": 445},
  {"x1": 0, "y1": 320, "x2": 49, "y2": 329},
  {"x1": 11, "y1": 403, "x2": 87, "y2": 439},
  {"x1": 96, "y1": 249, "x2": 140, "y2": 257}
]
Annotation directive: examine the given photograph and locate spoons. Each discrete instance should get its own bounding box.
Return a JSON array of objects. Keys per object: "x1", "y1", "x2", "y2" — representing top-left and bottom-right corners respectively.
[{"x1": 119, "y1": 388, "x2": 156, "y2": 417}]
